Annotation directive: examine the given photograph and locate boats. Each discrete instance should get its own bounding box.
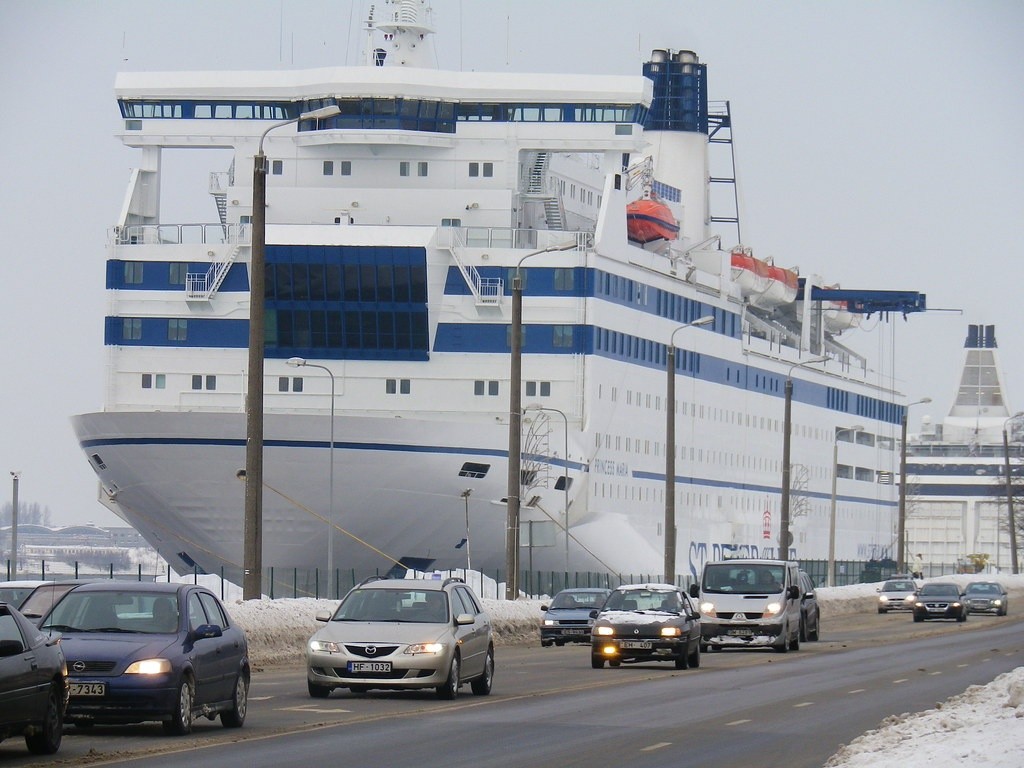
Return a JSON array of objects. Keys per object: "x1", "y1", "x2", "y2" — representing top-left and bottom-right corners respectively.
[
  {"x1": 820, "y1": 285, "x2": 865, "y2": 331},
  {"x1": 730, "y1": 245, "x2": 800, "y2": 309}
]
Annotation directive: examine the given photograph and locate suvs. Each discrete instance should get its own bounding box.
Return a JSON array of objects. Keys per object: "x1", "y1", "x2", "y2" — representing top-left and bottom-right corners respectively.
[
  {"x1": 307, "y1": 577, "x2": 494, "y2": 702},
  {"x1": 690, "y1": 559, "x2": 820, "y2": 653}
]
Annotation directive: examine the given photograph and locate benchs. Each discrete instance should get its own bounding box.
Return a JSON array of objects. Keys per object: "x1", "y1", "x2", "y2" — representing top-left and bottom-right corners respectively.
[
  {"x1": 401, "y1": 608, "x2": 427, "y2": 619},
  {"x1": 117, "y1": 617, "x2": 201, "y2": 632}
]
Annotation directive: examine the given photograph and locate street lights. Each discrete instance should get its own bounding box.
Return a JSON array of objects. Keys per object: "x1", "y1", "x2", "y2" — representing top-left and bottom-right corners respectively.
[
  {"x1": 777, "y1": 354, "x2": 832, "y2": 562},
  {"x1": 664, "y1": 315, "x2": 715, "y2": 585},
  {"x1": 827, "y1": 425, "x2": 865, "y2": 585},
  {"x1": 1003, "y1": 412, "x2": 1024, "y2": 573},
  {"x1": 242, "y1": 104, "x2": 343, "y2": 598},
  {"x1": 506, "y1": 238, "x2": 578, "y2": 599},
  {"x1": 896, "y1": 396, "x2": 932, "y2": 576}
]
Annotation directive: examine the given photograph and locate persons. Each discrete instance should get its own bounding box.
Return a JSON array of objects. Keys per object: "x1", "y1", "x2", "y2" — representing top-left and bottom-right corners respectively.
[
  {"x1": 564, "y1": 597, "x2": 571, "y2": 605},
  {"x1": 667, "y1": 594, "x2": 676, "y2": 609},
  {"x1": 913, "y1": 554, "x2": 923, "y2": 580},
  {"x1": 153, "y1": 598, "x2": 176, "y2": 625},
  {"x1": 592, "y1": 594, "x2": 604, "y2": 607},
  {"x1": 762, "y1": 570, "x2": 779, "y2": 588},
  {"x1": 427, "y1": 596, "x2": 445, "y2": 618}
]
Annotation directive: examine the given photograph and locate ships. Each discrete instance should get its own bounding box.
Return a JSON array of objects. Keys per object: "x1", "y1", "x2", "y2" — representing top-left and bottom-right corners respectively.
[{"x1": 69, "y1": 1, "x2": 961, "y2": 600}]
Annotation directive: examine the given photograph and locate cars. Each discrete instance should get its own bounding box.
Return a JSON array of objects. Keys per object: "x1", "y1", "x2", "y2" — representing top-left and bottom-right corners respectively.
[
  {"x1": 875, "y1": 579, "x2": 1008, "y2": 623},
  {"x1": 1, "y1": 575, "x2": 253, "y2": 756},
  {"x1": 539, "y1": 582, "x2": 702, "y2": 671}
]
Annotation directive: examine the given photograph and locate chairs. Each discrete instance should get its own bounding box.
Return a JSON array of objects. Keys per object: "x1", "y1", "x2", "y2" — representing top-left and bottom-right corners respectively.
[
  {"x1": 657, "y1": 600, "x2": 670, "y2": 611},
  {"x1": 365, "y1": 594, "x2": 400, "y2": 619},
  {"x1": 733, "y1": 573, "x2": 750, "y2": 587},
  {"x1": 622, "y1": 600, "x2": 637, "y2": 610},
  {"x1": 887, "y1": 584, "x2": 895, "y2": 589},
  {"x1": 81, "y1": 597, "x2": 119, "y2": 630},
  {"x1": 927, "y1": 589, "x2": 937, "y2": 595}
]
